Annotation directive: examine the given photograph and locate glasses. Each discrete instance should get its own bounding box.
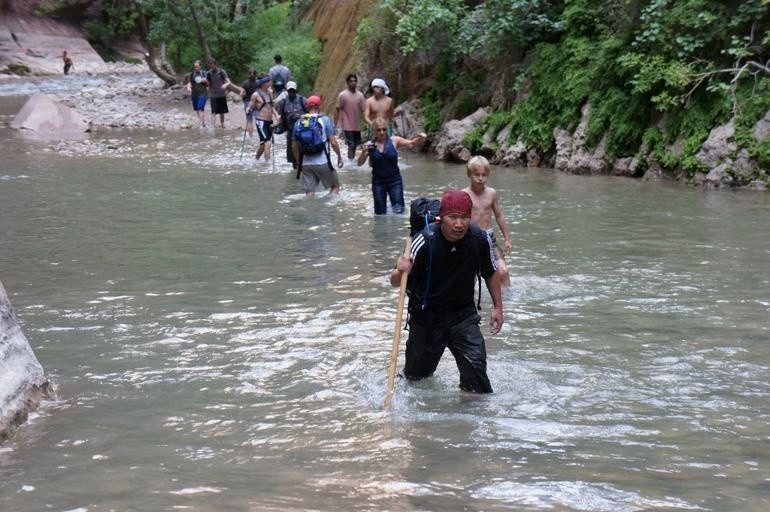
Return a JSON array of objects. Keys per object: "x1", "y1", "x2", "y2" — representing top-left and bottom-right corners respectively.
[{"x1": 376, "y1": 127, "x2": 387, "y2": 132}]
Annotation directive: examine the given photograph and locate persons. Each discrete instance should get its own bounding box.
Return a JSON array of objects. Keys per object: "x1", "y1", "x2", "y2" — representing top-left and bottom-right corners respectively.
[
  {"x1": 203, "y1": 58, "x2": 231, "y2": 129},
  {"x1": 56, "y1": 50, "x2": 74, "y2": 76},
  {"x1": 239, "y1": 54, "x2": 427, "y2": 216},
  {"x1": 181, "y1": 60, "x2": 208, "y2": 130},
  {"x1": 460, "y1": 154, "x2": 512, "y2": 290},
  {"x1": 389, "y1": 189, "x2": 504, "y2": 395}
]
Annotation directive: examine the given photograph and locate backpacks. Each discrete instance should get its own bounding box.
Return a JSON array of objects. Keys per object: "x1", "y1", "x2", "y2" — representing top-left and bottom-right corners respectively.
[
  {"x1": 295, "y1": 113, "x2": 324, "y2": 155},
  {"x1": 409, "y1": 197, "x2": 441, "y2": 237},
  {"x1": 271, "y1": 66, "x2": 286, "y2": 92}
]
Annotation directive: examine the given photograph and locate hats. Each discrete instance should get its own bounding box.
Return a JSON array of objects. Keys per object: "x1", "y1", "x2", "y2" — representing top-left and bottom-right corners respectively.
[
  {"x1": 307, "y1": 95, "x2": 321, "y2": 107},
  {"x1": 285, "y1": 81, "x2": 297, "y2": 91},
  {"x1": 371, "y1": 78, "x2": 390, "y2": 94}
]
